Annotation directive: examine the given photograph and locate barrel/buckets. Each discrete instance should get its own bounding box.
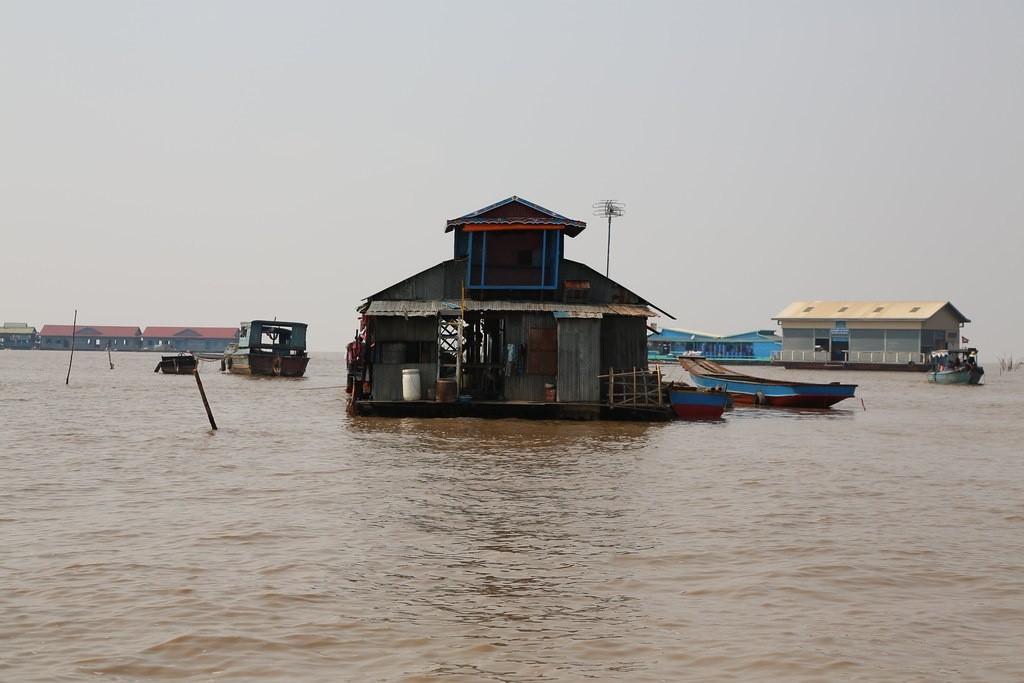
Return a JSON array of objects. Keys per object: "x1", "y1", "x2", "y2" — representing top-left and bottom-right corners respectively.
[
  {"x1": 436, "y1": 378, "x2": 457, "y2": 403},
  {"x1": 402, "y1": 369, "x2": 421, "y2": 401}
]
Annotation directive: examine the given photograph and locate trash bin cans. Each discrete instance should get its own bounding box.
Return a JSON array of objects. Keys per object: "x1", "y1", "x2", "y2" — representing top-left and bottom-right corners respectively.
[{"x1": 402, "y1": 369, "x2": 421, "y2": 401}]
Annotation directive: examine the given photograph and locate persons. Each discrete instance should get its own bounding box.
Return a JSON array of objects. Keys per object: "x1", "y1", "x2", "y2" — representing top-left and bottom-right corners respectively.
[{"x1": 929, "y1": 354, "x2": 958, "y2": 372}]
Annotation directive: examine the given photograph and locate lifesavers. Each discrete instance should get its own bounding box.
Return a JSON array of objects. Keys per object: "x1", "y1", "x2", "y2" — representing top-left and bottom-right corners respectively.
[
  {"x1": 221, "y1": 358, "x2": 232, "y2": 370},
  {"x1": 349, "y1": 341, "x2": 362, "y2": 357},
  {"x1": 752, "y1": 391, "x2": 766, "y2": 405},
  {"x1": 908, "y1": 360, "x2": 915, "y2": 368}
]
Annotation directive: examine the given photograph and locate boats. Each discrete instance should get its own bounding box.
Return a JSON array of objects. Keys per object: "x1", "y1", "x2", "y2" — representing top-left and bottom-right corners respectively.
[
  {"x1": 667, "y1": 386, "x2": 727, "y2": 421},
  {"x1": 160, "y1": 353, "x2": 199, "y2": 375},
  {"x1": 926, "y1": 347, "x2": 984, "y2": 385},
  {"x1": 5, "y1": 342, "x2": 33, "y2": 350},
  {"x1": 648, "y1": 351, "x2": 678, "y2": 364},
  {"x1": 222, "y1": 319, "x2": 310, "y2": 376},
  {"x1": 675, "y1": 353, "x2": 859, "y2": 408}
]
